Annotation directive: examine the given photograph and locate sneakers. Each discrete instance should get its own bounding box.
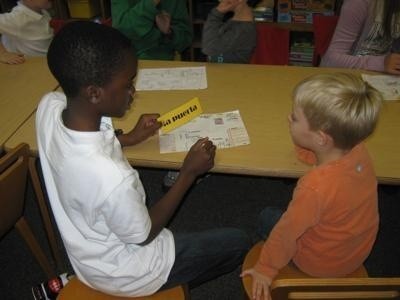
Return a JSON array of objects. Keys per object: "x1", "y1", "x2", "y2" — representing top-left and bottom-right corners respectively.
[{"x1": 30, "y1": 271, "x2": 71, "y2": 300}]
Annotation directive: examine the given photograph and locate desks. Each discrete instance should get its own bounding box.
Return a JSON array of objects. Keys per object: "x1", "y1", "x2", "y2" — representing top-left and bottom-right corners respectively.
[
  {"x1": 2, "y1": 51, "x2": 399, "y2": 188},
  {"x1": 189, "y1": 18, "x2": 314, "y2": 61}
]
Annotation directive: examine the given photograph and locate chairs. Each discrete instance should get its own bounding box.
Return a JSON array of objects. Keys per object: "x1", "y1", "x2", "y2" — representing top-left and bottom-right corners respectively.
[
  {"x1": 56, "y1": 277, "x2": 189, "y2": 299},
  {"x1": 247, "y1": 22, "x2": 292, "y2": 65},
  {"x1": 2, "y1": 143, "x2": 55, "y2": 299},
  {"x1": 311, "y1": 14, "x2": 341, "y2": 66},
  {"x1": 47, "y1": 16, "x2": 114, "y2": 32},
  {"x1": 271, "y1": 277, "x2": 399, "y2": 299}
]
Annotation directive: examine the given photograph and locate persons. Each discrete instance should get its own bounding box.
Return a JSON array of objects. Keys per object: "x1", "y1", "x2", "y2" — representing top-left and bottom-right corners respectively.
[
  {"x1": 318, "y1": 0, "x2": 400, "y2": 75},
  {"x1": 238, "y1": 70, "x2": 382, "y2": 300},
  {"x1": 1, "y1": 0, "x2": 55, "y2": 64},
  {"x1": 109, "y1": 0, "x2": 195, "y2": 61},
  {"x1": 200, "y1": 0, "x2": 262, "y2": 62},
  {"x1": 35, "y1": 19, "x2": 252, "y2": 298}
]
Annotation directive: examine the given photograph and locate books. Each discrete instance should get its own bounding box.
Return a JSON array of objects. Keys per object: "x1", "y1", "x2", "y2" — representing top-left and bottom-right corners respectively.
[{"x1": 251, "y1": 0, "x2": 334, "y2": 66}]
